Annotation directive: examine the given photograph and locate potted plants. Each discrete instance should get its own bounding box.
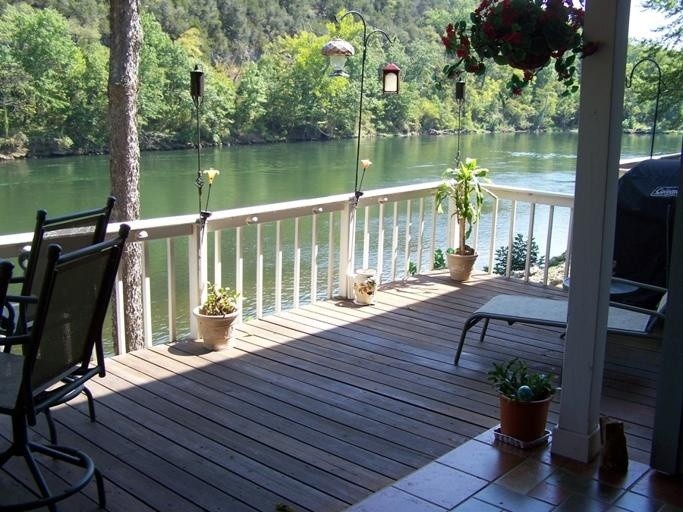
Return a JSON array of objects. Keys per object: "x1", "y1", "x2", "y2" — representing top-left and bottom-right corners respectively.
[
  {"x1": 192, "y1": 280, "x2": 240, "y2": 351},
  {"x1": 434, "y1": 156, "x2": 491, "y2": 281},
  {"x1": 486, "y1": 355, "x2": 561, "y2": 443}
]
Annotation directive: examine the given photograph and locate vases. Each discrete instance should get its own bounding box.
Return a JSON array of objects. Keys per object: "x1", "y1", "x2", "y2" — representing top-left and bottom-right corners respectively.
[
  {"x1": 500, "y1": 40, "x2": 552, "y2": 69},
  {"x1": 353, "y1": 269, "x2": 378, "y2": 305}
]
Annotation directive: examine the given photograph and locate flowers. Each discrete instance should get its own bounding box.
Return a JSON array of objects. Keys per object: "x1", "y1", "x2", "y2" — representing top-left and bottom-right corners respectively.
[
  {"x1": 354, "y1": 159, "x2": 372, "y2": 207},
  {"x1": 434, "y1": 1, "x2": 601, "y2": 97},
  {"x1": 201, "y1": 167, "x2": 220, "y2": 242}
]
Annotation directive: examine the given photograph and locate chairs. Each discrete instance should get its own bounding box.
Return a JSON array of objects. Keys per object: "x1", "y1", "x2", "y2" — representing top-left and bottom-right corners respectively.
[{"x1": 453, "y1": 277, "x2": 669, "y2": 365}]
